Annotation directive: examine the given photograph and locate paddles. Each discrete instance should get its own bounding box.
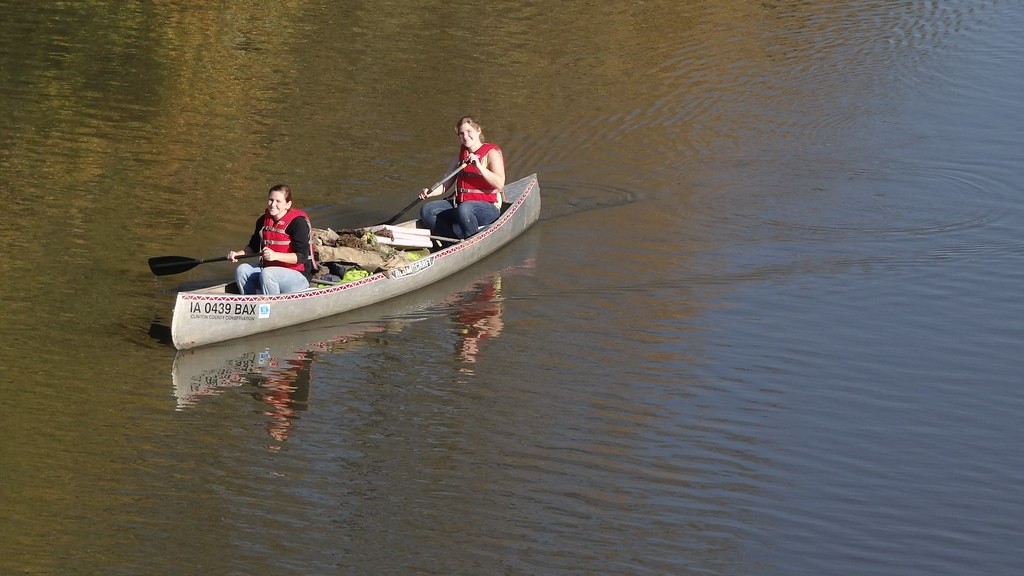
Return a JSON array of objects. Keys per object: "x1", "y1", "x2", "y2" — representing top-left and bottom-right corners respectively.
[
  {"x1": 148, "y1": 253, "x2": 263, "y2": 276},
  {"x1": 373, "y1": 159, "x2": 469, "y2": 226}
]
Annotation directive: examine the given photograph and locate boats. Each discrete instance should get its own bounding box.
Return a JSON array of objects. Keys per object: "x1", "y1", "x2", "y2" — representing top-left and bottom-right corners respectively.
[
  {"x1": 170, "y1": 173, "x2": 541, "y2": 353},
  {"x1": 171, "y1": 233, "x2": 538, "y2": 412}
]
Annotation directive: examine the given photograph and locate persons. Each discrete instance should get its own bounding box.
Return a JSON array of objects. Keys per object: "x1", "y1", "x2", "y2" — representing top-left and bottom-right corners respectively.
[
  {"x1": 419, "y1": 115, "x2": 506, "y2": 244},
  {"x1": 227, "y1": 185, "x2": 317, "y2": 295}
]
